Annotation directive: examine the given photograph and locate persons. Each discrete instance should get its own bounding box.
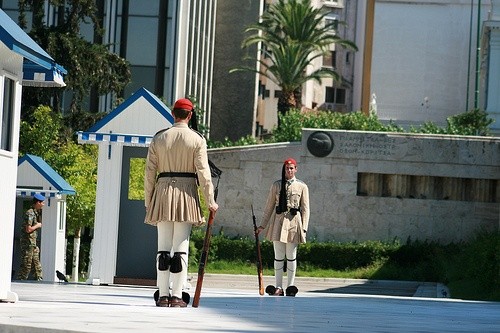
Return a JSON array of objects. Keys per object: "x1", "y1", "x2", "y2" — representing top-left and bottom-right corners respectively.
[
  {"x1": 17, "y1": 192, "x2": 46, "y2": 282},
  {"x1": 256, "y1": 158, "x2": 312, "y2": 296},
  {"x1": 144, "y1": 99, "x2": 219, "y2": 308}
]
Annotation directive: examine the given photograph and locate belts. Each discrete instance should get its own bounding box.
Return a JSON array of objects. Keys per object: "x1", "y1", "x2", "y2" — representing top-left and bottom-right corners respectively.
[
  {"x1": 277, "y1": 207, "x2": 301, "y2": 211},
  {"x1": 159, "y1": 171, "x2": 196, "y2": 177}
]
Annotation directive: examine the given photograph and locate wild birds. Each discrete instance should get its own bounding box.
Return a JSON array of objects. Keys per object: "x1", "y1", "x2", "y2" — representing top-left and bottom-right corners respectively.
[{"x1": 56, "y1": 270, "x2": 68, "y2": 283}]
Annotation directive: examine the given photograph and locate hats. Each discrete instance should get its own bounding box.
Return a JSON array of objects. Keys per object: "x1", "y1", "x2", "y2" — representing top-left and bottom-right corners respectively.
[
  {"x1": 174, "y1": 98, "x2": 193, "y2": 111},
  {"x1": 34, "y1": 193, "x2": 46, "y2": 201},
  {"x1": 284, "y1": 158, "x2": 296, "y2": 164}
]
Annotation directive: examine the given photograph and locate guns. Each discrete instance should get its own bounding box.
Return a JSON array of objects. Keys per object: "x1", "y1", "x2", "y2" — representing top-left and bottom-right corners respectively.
[
  {"x1": 250, "y1": 205, "x2": 265, "y2": 295},
  {"x1": 193, "y1": 179, "x2": 221, "y2": 309}
]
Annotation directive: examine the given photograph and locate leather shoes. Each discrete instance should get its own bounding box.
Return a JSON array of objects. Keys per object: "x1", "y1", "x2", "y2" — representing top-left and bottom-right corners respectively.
[
  {"x1": 286, "y1": 292, "x2": 295, "y2": 296},
  {"x1": 269, "y1": 287, "x2": 284, "y2": 295},
  {"x1": 156, "y1": 296, "x2": 170, "y2": 307},
  {"x1": 170, "y1": 296, "x2": 187, "y2": 307}
]
